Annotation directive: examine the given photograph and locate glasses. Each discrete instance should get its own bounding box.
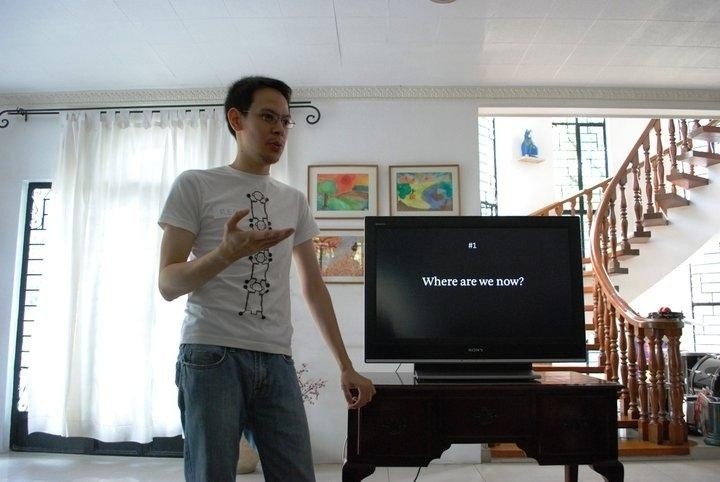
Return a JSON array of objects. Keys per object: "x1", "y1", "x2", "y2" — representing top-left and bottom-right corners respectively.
[{"x1": 241, "y1": 110, "x2": 296, "y2": 129}]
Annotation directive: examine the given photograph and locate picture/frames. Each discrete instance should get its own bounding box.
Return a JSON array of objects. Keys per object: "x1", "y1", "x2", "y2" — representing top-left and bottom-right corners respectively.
[{"x1": 304, "y1": 163, "x2": 462, "y2": 287}]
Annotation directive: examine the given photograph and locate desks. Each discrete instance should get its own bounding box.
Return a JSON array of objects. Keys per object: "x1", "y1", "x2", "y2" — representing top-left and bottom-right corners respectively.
[{"x1": 338, "y1": 370, "x2": 631, "y2": 482}]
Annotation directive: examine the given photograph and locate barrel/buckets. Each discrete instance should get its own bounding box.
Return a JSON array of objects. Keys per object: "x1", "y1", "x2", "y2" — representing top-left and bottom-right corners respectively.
[{"x1": 703, "y1": 396, "x2": 720, "y2": 446}]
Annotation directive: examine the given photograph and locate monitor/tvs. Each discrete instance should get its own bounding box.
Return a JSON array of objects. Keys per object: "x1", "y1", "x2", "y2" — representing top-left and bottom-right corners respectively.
[{"x1": 364, "y1": 215, "x2": 587, "y2": 379}]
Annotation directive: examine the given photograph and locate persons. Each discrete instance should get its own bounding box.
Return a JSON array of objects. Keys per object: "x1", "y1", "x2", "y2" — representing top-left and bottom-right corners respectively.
[{"x1": 158, "y1": 76, "x2": 376, "y2": 482}]
